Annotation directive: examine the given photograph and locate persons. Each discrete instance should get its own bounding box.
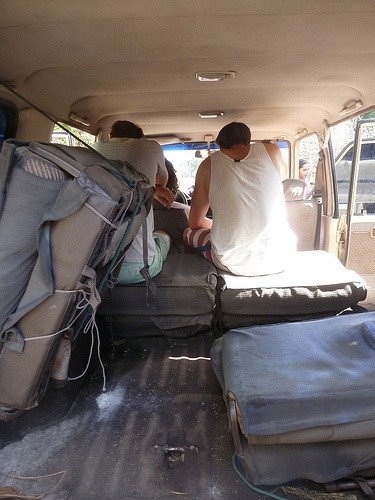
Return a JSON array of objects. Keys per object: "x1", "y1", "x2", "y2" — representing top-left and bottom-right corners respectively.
[
  {"x1": 299, "y1": 159, "x2": 312, "y2": 200},
  {"x1": 184, "y1": 121, "x2": 297, "y2": 277},
  {"x1": 90, "y1": 120, "x2": 176, "y2": 285}
]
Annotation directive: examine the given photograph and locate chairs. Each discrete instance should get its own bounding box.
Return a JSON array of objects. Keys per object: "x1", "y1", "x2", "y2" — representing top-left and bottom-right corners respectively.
[{"x1": 283, "y1": 179, "x2": 318, "y2": 251}]
